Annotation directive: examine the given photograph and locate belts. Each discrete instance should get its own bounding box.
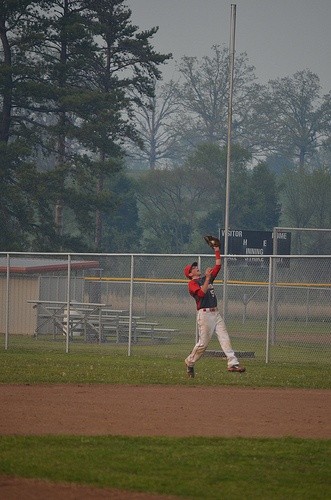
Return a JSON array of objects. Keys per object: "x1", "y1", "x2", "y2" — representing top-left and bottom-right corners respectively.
[{"x1": 203, "y1": 308, "x2": 218, "y2": 311}]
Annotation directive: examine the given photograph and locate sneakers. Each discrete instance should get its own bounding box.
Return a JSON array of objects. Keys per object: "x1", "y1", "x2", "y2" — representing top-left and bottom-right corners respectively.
[
  {"x1": 228, "y1": 365, "x2": 245, "y2": 371},
  {"x1": 186, "y1": 364, "x2": 194, "y2": 378}
]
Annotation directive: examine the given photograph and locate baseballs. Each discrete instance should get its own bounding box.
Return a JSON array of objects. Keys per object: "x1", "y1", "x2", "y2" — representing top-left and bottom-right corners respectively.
[{"x1": 211, "y1": 240, "x2": 216, "y2": 244}]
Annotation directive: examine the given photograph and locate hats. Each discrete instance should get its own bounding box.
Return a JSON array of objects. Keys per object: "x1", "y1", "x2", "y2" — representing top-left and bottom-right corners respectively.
[{"x1": 184, "y1": 262, "x2": 197, "y2": 278}]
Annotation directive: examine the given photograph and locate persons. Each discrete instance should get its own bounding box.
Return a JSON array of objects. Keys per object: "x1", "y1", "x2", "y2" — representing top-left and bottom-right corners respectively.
[{"x1": 184, "y1": 236, "x2": 246, "y2": 379}]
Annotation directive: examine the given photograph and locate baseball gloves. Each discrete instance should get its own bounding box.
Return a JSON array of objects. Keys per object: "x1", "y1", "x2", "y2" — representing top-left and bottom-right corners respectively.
[{"x1": 204, "y1": 236, "x2": 222, "y2": 248}]
